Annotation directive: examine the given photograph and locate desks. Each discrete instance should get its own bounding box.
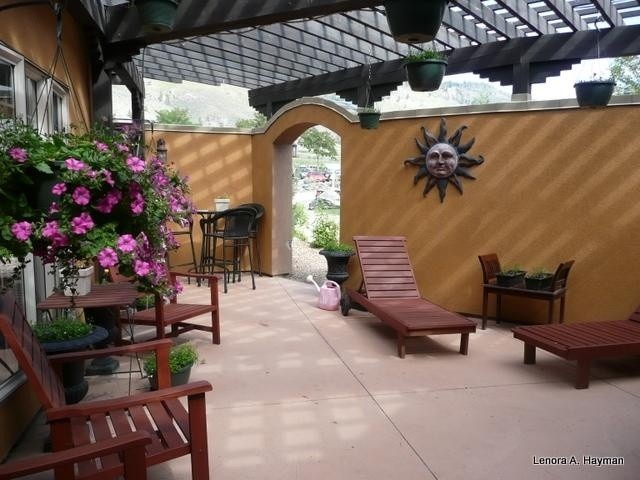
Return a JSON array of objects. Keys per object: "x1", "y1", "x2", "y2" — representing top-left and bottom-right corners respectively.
[{"x1": 36, "y1": 284, "x2": 140, "y2": 376}]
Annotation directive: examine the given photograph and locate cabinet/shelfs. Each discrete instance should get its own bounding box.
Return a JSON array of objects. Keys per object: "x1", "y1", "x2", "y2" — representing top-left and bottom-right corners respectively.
[{"x1": 477, "y1": 253, "x2": 575, "y2": 332}]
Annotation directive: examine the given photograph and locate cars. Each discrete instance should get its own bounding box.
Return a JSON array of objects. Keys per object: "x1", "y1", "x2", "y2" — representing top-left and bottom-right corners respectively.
[{"x1": 295, "y1": 162, "x2": 341, "y2": 190}]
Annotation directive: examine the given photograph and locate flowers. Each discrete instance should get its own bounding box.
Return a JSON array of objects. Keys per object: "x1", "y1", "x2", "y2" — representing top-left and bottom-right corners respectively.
[
  {"x1": 216, "y1": 192, "x2": 231, "y2": 198},
  {"x1": 0, "y1": 116, "x2": 197, "y2": 298}
]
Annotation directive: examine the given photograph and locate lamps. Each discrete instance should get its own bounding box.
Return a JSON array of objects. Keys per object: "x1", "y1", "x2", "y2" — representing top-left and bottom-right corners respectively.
[{"x1": 155, "y1": 137, "x2": 168, "y2": 164}]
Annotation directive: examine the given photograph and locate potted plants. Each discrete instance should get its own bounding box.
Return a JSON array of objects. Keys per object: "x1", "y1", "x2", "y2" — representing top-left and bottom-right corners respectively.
[
  {"x1": 28, "y1": 317, "x2": 109, "y2": 406},
  {"x1": 143, "y1": 338, "x2": 198, "y2": 392},
  {"x1": 572, "y1": 79, "x2": 617, "y2": 108},
  {"x1": 524, "y1": 263, "x2": 555, "y2": 290},
  {"x1": 493, "y1": 261, "x2": 528, "y2": 287},
  {"x1": 404, "y1": 50, "x2": 449, "y2": 92},
  {"x1": 318, "y1": 240, "x2": 357, "y2": 290},
  {"x1": 357, "y1": 109, "x2": 380, "y2": 130}
]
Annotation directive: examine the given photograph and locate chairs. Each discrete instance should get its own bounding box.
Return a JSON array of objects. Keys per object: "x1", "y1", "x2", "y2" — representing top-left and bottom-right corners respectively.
[
  {"x1": 510, "y1": 304, "x2": 640, "y2": 389},
  {"x1": 343, "y1": 234, "x2": 477, "y2": 360},
  {"x1": 0, "y1": 430, "x2": 153, "y2": 480},
  {"x1": 0, "y1": 296, "x2": 214, "y2": 480},
  {"x1": 164, "y1": 202, "x2": 265, "y2": 293},
  {"x1": 107, "y1": 260, "x2": 220, "y2": 344}
]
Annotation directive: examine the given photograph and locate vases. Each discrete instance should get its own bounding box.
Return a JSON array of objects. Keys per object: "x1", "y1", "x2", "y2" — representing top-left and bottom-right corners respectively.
[
  {"x1": 214, "y1": 199, "x2": 230, "y2": 211},
  {"x1": 59, "y1": 266, "x2": 94, "y2": 296},
  {"x1": 380, "y1": 1, "x2": 449, "y2": 45},
  {"x1": 134, "y1": 0, "x2": 182, "y2": 34}
]
